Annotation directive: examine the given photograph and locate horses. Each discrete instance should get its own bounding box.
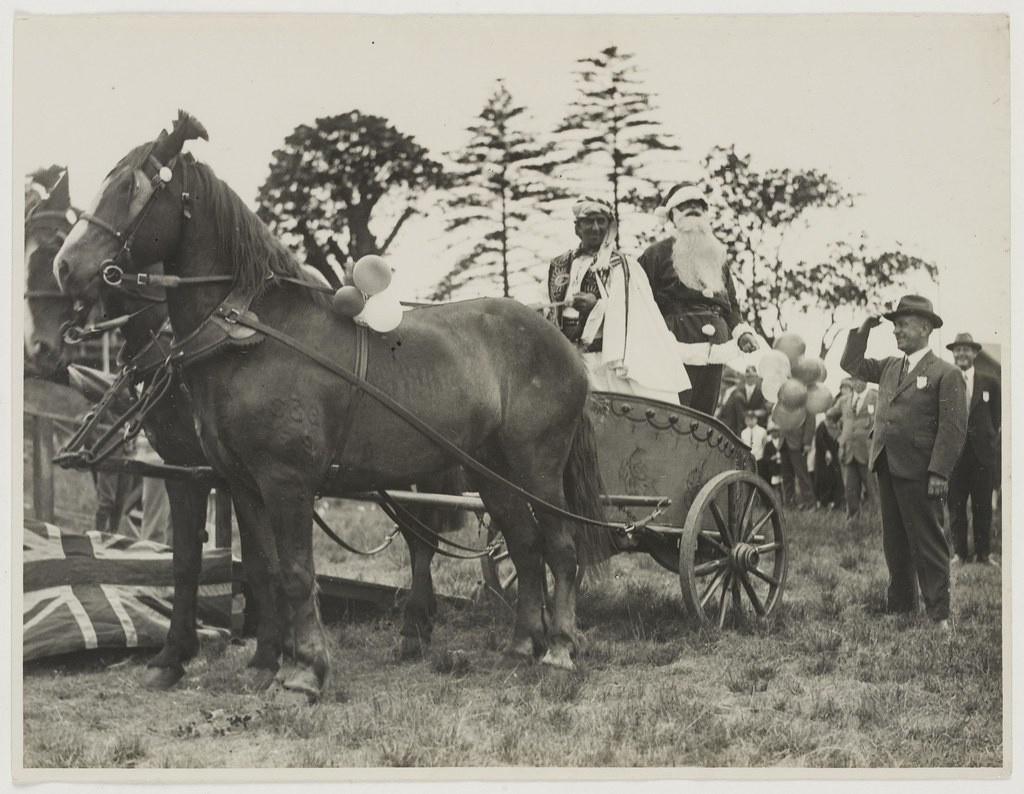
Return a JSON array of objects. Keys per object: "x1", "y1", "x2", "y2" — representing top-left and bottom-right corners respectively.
[{"x1": 24, "y1": 109, "x2": 618, "y2": 709}]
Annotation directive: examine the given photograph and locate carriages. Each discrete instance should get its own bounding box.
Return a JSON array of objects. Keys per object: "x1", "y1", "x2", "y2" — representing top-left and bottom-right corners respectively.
[{"x1": 23, "y1": 109, "x2": 790, "y2": 710}]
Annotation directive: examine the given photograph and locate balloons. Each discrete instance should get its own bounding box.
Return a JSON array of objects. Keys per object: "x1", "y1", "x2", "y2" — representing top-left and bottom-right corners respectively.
[
  {"x1": 758, "y1": 329, "x2": 834, "y2": 431},
  {"x1": 364, "y1": 294, "x2": 404, "y2": 332},
  {"x1": 352, "y1": 254, "x2": 393, "y2": 296},
  {"x1": 332, "y1": 285, "x2": 366, "y2": 319}
]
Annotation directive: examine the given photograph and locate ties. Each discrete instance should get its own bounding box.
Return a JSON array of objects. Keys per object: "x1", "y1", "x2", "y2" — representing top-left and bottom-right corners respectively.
[
  {"x1": 963, "y1": 374, "x2": 968, "y2": 391},
  {"x1": 900, "y1": 358, "x2": 910, "y2": 383},
  {"x1": 750, "y1": 431, "x2": 753, "y2": 450},
  {"x1": 852, "y1": 398, "x2": 859, "y2": 416}
]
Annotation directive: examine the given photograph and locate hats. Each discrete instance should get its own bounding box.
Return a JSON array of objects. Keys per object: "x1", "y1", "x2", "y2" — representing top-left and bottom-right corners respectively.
[
  {"x1": 573, "y1": 194, "x2": 617, "y2": 272},
  {"x1": 946, "y1": 333, "x2": 981, "y2": 351},
  {"x1": 883, "y1": 295, "x2": 943, "y2": 328},
  {"x1": 767, "y1": 425, "x2": 780, "y2": 434},
  {"x1": 722, "y1": 369, "x2": 741, "y2": 384},
  {"x1": 653, "y1": 181, "x2": 709, "y2": 225}
]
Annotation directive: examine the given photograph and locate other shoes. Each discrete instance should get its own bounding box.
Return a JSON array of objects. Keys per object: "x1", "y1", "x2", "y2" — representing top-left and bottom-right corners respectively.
[
  {"x1": 951, "y1": 555, "x2": 964, "y2": 566},
  {"x1": 977, "y1": 557, "x2": 999, "y2": 568}
]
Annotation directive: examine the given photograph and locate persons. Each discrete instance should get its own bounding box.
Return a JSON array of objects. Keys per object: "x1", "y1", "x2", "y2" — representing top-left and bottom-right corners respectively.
[
  {"x1": 636, "y1": 181, "x2": 759, "y2": 416},
  {"x1": 715, "y1": 364, "x2": 883, "y2": 520},
  {"x1": 548, "y1": 194, "x2": 656, "y2": 398},
  {"x1": 84, "y1": 367, "x2": 175, "y2": 546},
  {"x1": 944, "y1": 332, "x2": 1002, "y2": 570},
  {"x1": 839, "y1": 294, "x2": 969, "y2": 632}
]
